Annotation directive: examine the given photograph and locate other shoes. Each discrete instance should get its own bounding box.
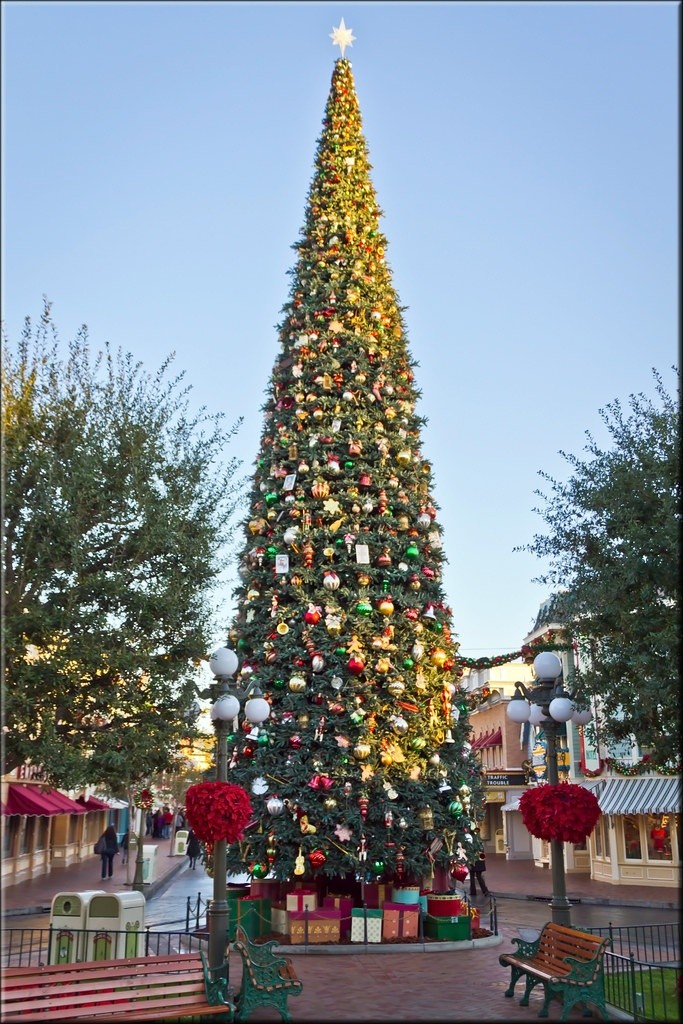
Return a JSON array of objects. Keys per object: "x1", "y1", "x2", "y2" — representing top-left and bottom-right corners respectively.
[
  {"x1": 189, "y1": 866, "x2": 196, "y2": 870},
  {"x1": 122, "y1": 862, "x2": 129, "y2": 865},
  {"x1": 102, "y1": 876, "x2": 113, "y2": 881},
  {"x1": 468, "y1": 891, "x2": 490, "y2": 896}
]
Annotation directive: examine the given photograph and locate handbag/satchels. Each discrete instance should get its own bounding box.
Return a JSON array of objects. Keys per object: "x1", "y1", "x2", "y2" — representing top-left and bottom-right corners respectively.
[{"x1": 94, "y1": 838, "x2": 106, "y2": 853}]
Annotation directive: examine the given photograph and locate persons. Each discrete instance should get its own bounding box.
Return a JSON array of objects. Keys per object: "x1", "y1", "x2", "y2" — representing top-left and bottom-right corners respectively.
[
  {"x1": 145, "y1": 807, "x2": 188, "y2": 841},
  {"x1": 120, "y1": 828, "x2": 129, "y2": 865},
  {"x1": 97, "y1": 825, "x2": 118, "y2": 880},
  {"x1": 185, "y1": 828, "x2": 201, "y2": 870},
  {"x1": 468, "y1": 849, "x2": 493, "y2": 897}
]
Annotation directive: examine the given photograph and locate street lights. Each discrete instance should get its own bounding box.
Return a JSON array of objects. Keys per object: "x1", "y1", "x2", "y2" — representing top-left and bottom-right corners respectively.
[
  {"x1": 505, "y1": 650, "x2": 591, "y2": 928},
  {"x1": 178, "y1": 645, "x2": 270, "y2": 984}
]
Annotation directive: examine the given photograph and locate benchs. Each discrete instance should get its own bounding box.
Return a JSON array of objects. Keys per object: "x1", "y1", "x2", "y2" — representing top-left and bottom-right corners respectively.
[{"x1": 497, "y1": 921, "x2": 613, "y2": 1023}]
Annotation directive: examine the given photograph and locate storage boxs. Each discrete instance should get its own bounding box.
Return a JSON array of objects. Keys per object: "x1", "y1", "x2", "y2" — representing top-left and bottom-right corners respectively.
[{"x1": 223, "y1": 870, "x2": 482, "y2": 943}]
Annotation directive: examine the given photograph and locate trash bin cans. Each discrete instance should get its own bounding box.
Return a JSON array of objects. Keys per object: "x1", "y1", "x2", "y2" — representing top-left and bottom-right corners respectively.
[
  {"x1": 141, "y1": 844, "x2": 159, "y2": 885},
  {"x1": 46, "y1": 890, "x2": 106, "y2": 975},
  {"x1": 83, "y1": 890, "x2": 146, "y2": 977},
  {"x1": 174, "y1": 830, "x2": 190, "y2": 856}
]
